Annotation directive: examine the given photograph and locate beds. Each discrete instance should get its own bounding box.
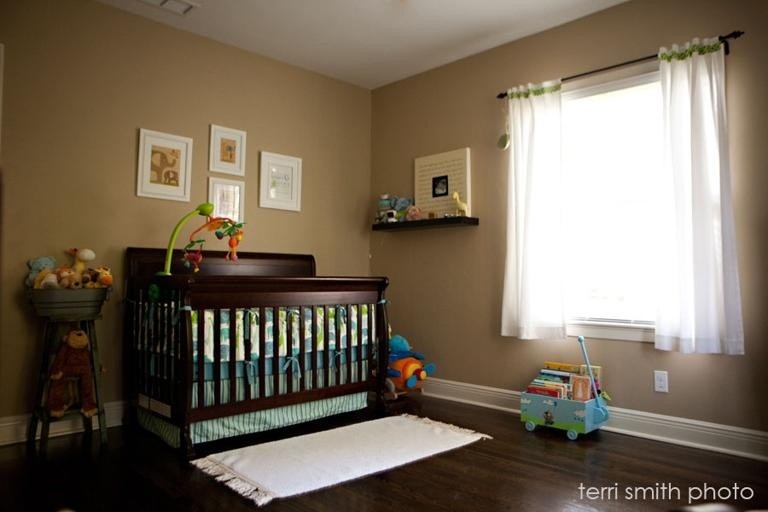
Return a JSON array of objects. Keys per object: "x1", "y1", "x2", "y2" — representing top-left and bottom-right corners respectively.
[{"x1": 122, "y1": 247, "x2": 389, "y2": 463}]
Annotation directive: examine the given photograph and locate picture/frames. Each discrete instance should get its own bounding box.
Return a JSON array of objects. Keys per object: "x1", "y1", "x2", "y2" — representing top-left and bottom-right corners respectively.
[{"x1": 134, "y1": 123, "x2": 304, "y2": 230}]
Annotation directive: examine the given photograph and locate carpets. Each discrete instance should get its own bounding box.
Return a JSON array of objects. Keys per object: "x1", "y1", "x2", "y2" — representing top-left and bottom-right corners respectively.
[{"x1": 187, "y1": 412, "x2": 494, "y2": 509}]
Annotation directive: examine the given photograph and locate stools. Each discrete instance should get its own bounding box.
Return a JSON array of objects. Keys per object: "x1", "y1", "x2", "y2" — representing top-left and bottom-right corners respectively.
[{"x1": 26, "y1": 314, "x2": 108, "y2": 449}]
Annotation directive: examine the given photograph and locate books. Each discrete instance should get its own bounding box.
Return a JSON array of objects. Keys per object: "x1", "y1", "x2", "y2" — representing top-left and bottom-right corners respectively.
[{"x1": 525, "y1": 359, "x2": 600, "y2": 401}]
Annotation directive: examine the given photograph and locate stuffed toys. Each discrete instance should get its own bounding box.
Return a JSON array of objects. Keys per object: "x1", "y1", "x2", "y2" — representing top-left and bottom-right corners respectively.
[
  {"x1": 44, "y1": 327, "x2": 100, "y2": 418},
  {"x1": 22, "y1": 245, "x2": 113, "y2": 290},
  {"x1": 373, "y1": 190, "x2": 468, "y2": 224},
  {"x1": 158, "y1": 203, "x2": 249, "y2": 275},
  {"x1": 383, "y1": 332, "x2": 439, "y2": 400}
]
